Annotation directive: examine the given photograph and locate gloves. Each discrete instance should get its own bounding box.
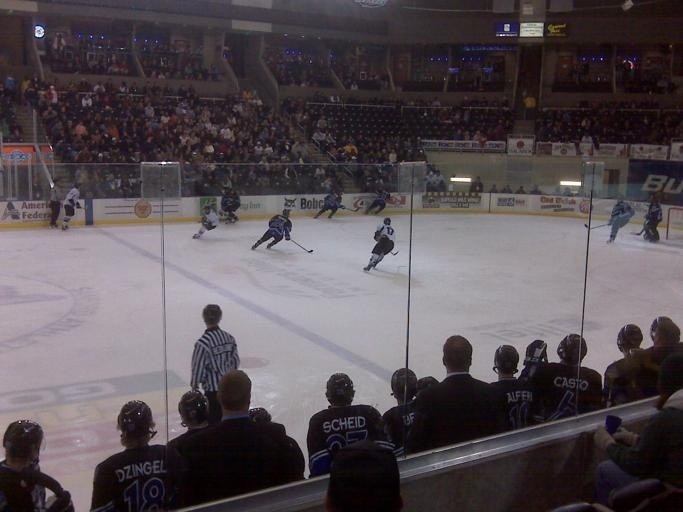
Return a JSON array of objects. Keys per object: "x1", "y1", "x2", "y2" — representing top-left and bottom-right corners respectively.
[
  {"x1": 524, "y1": 341, "x2": 546, "y2": 363},
  {"x1": 594, "y1": 426, "x2": 638, "y2": 452}
]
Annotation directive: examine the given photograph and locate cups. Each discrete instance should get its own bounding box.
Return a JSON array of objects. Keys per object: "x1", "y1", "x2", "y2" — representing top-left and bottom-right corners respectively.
[{"x1": 603, "y1": 415, "x2": 622, "y2": 434}]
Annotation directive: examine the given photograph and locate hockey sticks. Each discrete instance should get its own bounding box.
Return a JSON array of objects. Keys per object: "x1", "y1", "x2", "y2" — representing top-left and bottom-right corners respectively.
[
  {"x1": 630, "y1": 227, "x2": 645, "y2": 236},
  {"x1": 585, "y1": 223, "x2": 608, "y2": 229},
  {"x1": 345, "y1": 207, "x2": 360, "y2": 212},
  {"x1": 81, "y1": 205, "x2": 88, "y2": 210},
  {"x1": 390, "y1": 251, "x2": 399, "y2": 255},
  {"x1": 290, "y1": 239, "x2": 313, "y2": 253}
]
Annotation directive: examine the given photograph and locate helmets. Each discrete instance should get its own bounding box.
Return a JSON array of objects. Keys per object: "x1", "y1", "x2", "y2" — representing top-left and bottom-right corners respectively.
[
  {"x1": 558, "y1": 334, "x2": 587, "y2": 363},
  {"x1": 493, "y1": 345, "x2": 519, "y2": 372},
  {"x1": 178, "y1": 390, "x2": 210, "y2": 425},
  {"x1": 251, "y1": 407, "x2": 270, "y2": 424},
  {"x1": 391, "y1": 369, "x2": 416, "y2": 399},
  {"x1": 328, "y1": 374, "x2": 355, "y2": 404},
  {"x1": 4, "y1": 421, "x2": 45, "y2": 457},
  {"x1": 616, "y1": 325, "x2": 642, "y2": 350},
  {"x1": 118, "y1": 401, "x2": 153, "y2": 438}
]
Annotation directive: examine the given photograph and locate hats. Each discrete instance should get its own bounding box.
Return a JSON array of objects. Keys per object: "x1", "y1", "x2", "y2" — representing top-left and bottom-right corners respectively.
[{"x1": 328, "y1": 438, "x2": 401, "y2": 499}]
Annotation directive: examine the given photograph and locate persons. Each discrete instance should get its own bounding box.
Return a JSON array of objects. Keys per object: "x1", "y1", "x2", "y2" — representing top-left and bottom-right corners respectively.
[
  {"x1": 90, "y1": 370, "x2": 306, "y2": 512},
  {"x1": 642, "y1": 193, "x2": 663, "y2": 241},
  {"x1": 309, "y1": 314, "x2": 682, "y2": 512},
  {"x1": 61, "y1": 183, "x2": 82, "y2": 230},
  {"x1": 220, "y1": 187, "x2": 240, "y2": 222},
  {"x1": 1, "y1": 419, "x2": 75, "y2": 512},
  {"x1": 46, "y1": 177, "x2": 63, "y2": 229},
  {"x1": 363, "y1": 186, "x2": 390, "y2": 214},
  {"x1": 189, "y1": 304, "x2": 239, "y2": 422},
  {"x1": 250, "y1": 207, "x2": 292, "y2": 251},
  {"x1": 190, "y1": 205, "x2": 217, "y2": 238},
  {"x1": 362, "y1": 217, "x2": 394, "y2": 271},
  {"x1": 0, "y1": 30, "x2": 679, "y2": 203},
  {"x1": 606, "y1": 194, "x2": 635, "y2": 242},
  {"x1": 313, "y1": 190, "x2": 343, "y2": 219}
]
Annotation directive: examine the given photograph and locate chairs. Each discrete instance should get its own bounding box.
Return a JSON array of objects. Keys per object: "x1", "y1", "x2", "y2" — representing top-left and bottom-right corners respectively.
[
  {"x1": 531, "y1": 462, "x2": 683, "y2": 510},
  {"x1": 0, "y1": 29, "x2": 682, "y2": 200}
]
